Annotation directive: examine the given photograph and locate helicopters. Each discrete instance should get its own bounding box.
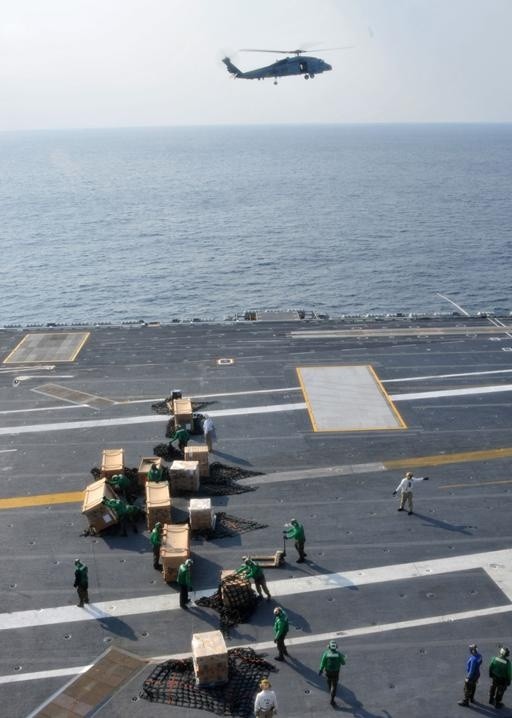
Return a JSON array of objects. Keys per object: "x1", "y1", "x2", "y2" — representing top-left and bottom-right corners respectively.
[{"x1": 221, "y1": 47, "x2": 332, "y2": 85}]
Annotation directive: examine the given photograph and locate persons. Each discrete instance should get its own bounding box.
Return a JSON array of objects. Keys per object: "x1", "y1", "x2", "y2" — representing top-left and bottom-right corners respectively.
[
  {"x1": 254, "y1": 679, "x2": 279, "y2": 718},
  {"x1": 457, "y1": 643, "x2": 483, "y2": 707},
  {"x1": 271, "y1": 606, "x2": 289, "y2": 661},
  {"x1": 102, "y1": 463, "x2": 162, "y2": 538},
  {"x1": 74, "y1": 559, "x2": 89, "y2": 608},
  {"x1": 236, "y1": 558, "x2": 272, "y2": 601},
  {"x1": 283, "y1": 518, "x2": 307, "y2": 563},
  {"x1": 203, "y1": 414, "x2": 214, "y2": 452},
  {"x1": 168, "y1": 424, "x2": 190, "y2": 451},
  {"x1": 489, "y1": 648, "x2": 512, "y2": 710},
  {"x1": 176, "y1": 558, "x2": 194, "y2": 609},
  {"x1": 319, "y1": 640, "x2": 347, "y2": 704},
  {"x1": 393, "y1": 472, "x2": 429, "y2": 515},
  {"x1": 150, "y1": 521, "x2": 163, "y2": 570}
]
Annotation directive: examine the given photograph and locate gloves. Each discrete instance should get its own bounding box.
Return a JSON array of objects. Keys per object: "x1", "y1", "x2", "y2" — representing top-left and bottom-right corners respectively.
[
  {"x1": 424, "y1": 477, "x2": 428, "y2": 480},
  {"x1": 392, "y1": 491, "x2": 397, "y2": 495}
]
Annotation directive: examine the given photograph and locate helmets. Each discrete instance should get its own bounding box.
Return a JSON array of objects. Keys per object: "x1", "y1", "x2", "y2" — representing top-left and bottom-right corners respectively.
[
  {"x1": 154, "y1": 523, "x2": 162, "y2": 529},
  {"x1": 112, "y1": 475, "x2": 119, "y2": 481},
  {"x1": 273, "y1": 607, "x2": 283, "y2": 615},
  {"x1": 291, "y1": 518, "x2": 298, "y2": 527},
  {"x1": 406, "y1": 472, "x2": 413, "y2": 476},
  {"x1": 185, "y1": 559, "x2": 193, "y2": 566},
  {"x1": 259, "y1": 679, "x2": 272, "y2": 689},
  {"x1": 499, "y1": 647, "x2": 510, "y2": 657},
  {"x1": 468, "y1": 643, "x2": 478, "y2": 655},
  {"x1": 328, "y1": 640, "x2": 338, "y2": 651},
  {"x1": 246, "y1": 560, "x2": 252, "y2": 566}
]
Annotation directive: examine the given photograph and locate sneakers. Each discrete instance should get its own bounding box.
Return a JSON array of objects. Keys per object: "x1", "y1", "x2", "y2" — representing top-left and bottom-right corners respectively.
[
  {"x1": 457, "y1": 697, "x2": 506, "y2": 711},
  {"x1": 275, "y1": 656, "x2": 284, "y2": 662}
]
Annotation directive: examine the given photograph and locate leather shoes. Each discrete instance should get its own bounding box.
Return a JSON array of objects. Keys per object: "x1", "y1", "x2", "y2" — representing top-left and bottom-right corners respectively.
[
  {"x1": 398, "y1": 509, "x2": 404, "y2": 511},
  {"x1": 408, "y1": 511, "x2": 412, "y2": 515}
]
